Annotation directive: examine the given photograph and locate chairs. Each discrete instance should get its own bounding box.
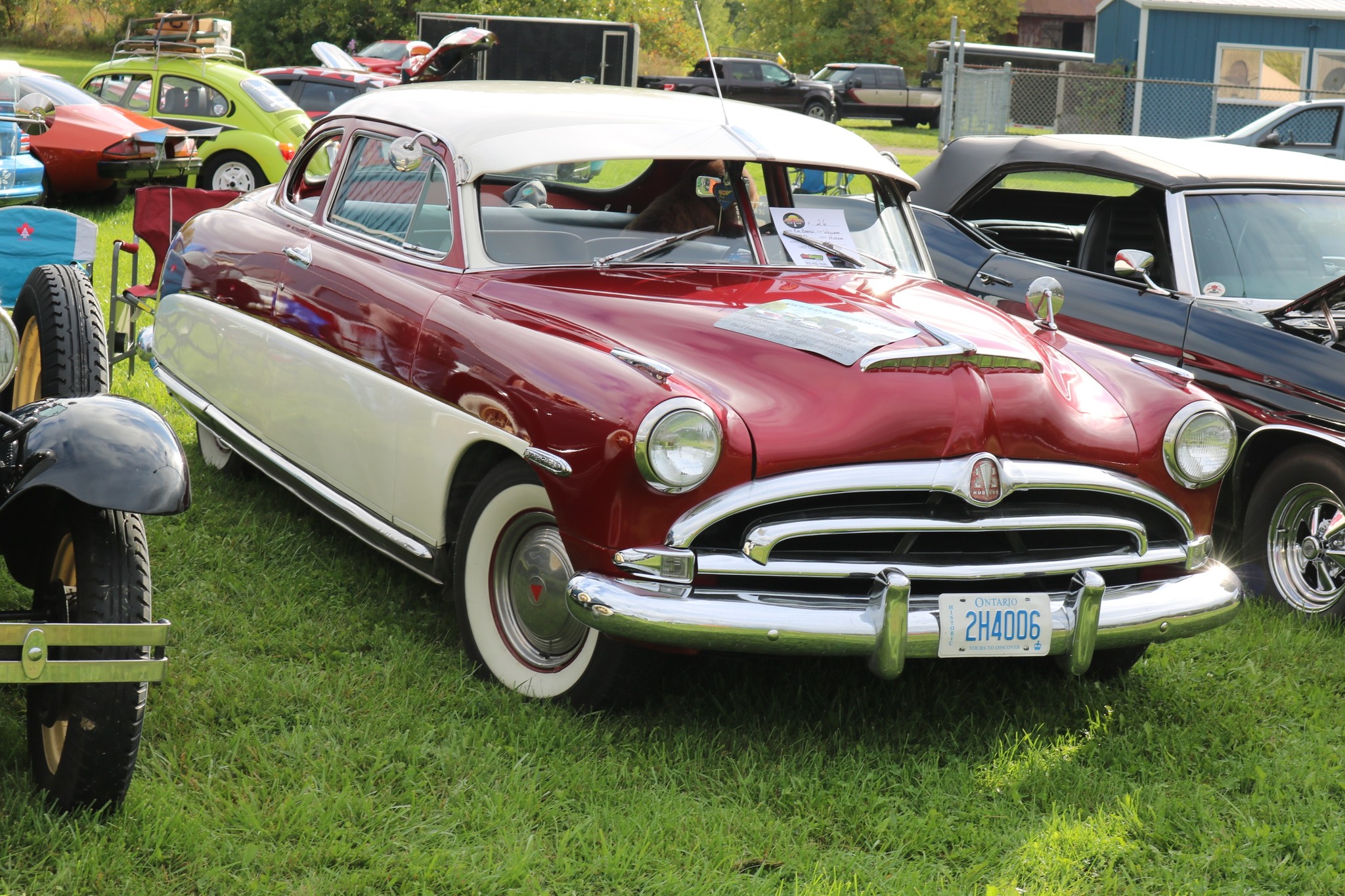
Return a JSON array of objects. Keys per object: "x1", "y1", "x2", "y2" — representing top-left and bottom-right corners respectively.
[
  {"x1": 173, "y1": 101, "x2": 174, "y2": 102},
  {"x1": 108, "y1": 185, "x2": 245, "y2": 391},
  {"x1": 187, "y1": 86, "x2": 207, "y2": 114},
  {"x1": 1078, "y1": 196, "x2": 1176, "y2": 290},
  {"x1": 163, "y1": 88, "x2": 185, "y2": 114}
]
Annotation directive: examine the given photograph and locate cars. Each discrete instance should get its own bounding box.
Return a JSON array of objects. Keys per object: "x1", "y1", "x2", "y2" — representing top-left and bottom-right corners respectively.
[
  {"x1": 0, "y1": 26, "x2": 500, "y2": 208},
  {"x1": 1182, "y1": 96, "x2": 1345, "y2": 161},
  {"x1": 0, "y1": 262, "x2": 193, "y2": 825},
  {"x1": 754, "y1": 133, "x2": 1345, "y2": 633},
  {"x1": 134, "y1": 75, "x2": 1243, "y2": 706}
]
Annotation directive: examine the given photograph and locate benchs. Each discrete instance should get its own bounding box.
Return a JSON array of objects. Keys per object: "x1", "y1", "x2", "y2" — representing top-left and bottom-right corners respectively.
[
  {"x1": 338, "y1": 200, "x2": 752, "y2": 265},
  {"x1": 970, "y1": 219, "x2": 1087, "y2": 267}
]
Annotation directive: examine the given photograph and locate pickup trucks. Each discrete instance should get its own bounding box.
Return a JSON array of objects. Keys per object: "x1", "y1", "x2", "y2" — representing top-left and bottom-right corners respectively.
[
  {"x1": 636, "y1": 56, "x2": 836, "y2": 123},
  {"x1": 808, "y1": 62, "x2": 957, "y2": 130}
]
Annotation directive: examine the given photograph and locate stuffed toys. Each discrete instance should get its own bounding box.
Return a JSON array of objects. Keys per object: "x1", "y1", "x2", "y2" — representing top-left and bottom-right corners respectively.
[{"x1": 625, "y1": 159, "x2": 758, "y2": 238}]
